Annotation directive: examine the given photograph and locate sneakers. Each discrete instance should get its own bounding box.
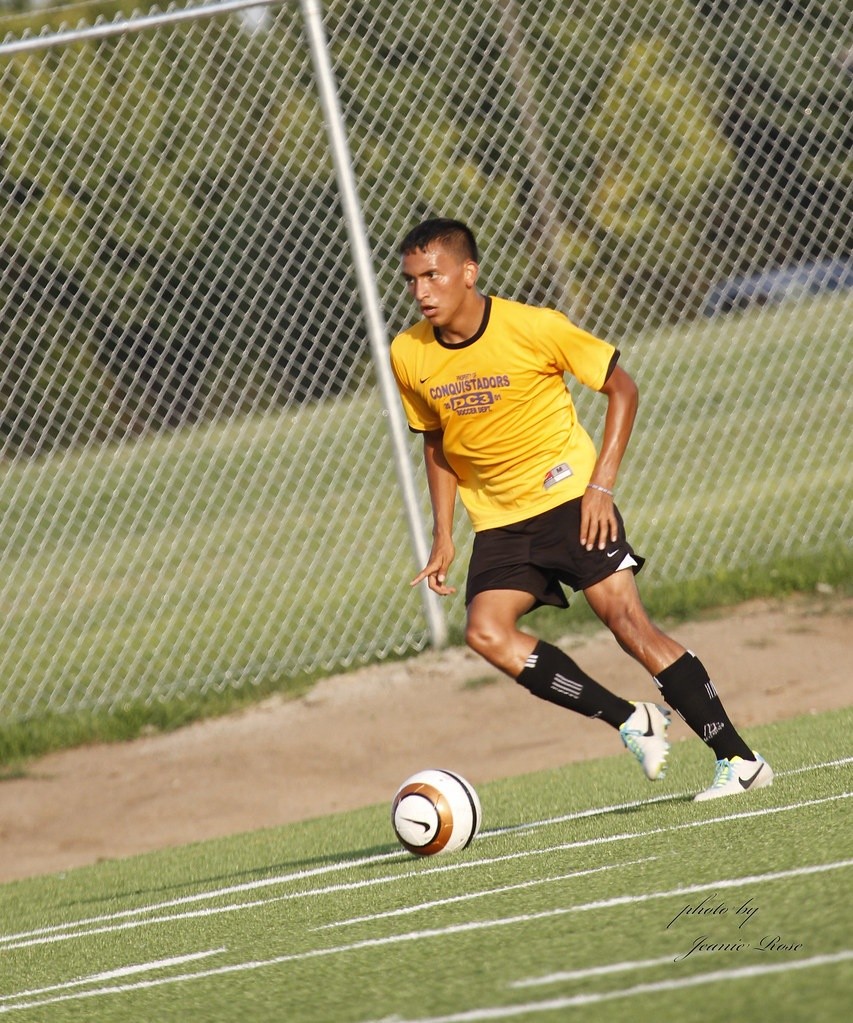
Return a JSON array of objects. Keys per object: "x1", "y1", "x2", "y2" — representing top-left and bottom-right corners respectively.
[
  {"x1": 695, "y1": 752, "x2": 774, "y2": 803},
  {"x1": 618, "y1": 701, "x2": 671, "y2": 780}
]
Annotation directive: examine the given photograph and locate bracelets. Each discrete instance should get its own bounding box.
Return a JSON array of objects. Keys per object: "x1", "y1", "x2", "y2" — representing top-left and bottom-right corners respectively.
[{"x1": 586, "y1": 481, "x2": 615, "y2": 499}]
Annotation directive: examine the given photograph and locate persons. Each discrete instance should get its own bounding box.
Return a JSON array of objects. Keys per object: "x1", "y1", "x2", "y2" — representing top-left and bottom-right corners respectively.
[{"x1": 387, "y1": 220, "x2": 779, "y2": 802}]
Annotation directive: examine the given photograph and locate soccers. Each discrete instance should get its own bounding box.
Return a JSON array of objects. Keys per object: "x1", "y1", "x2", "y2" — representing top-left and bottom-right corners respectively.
[{"x1": 389, "y1": 768, "x2": 483, "y2": 859}]
